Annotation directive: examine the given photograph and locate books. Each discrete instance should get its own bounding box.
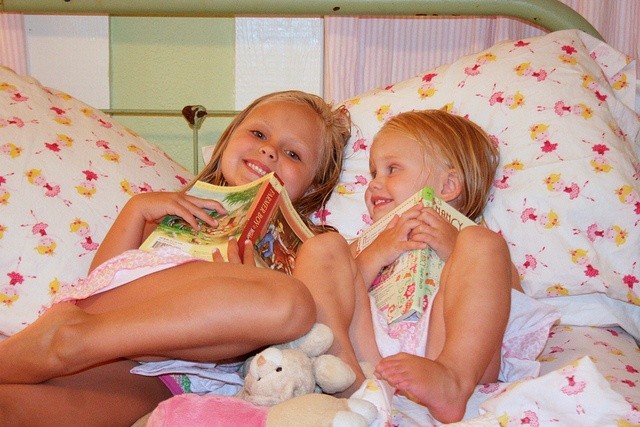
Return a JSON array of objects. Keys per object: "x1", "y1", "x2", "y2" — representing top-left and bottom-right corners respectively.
[
  {"x1": 137, "y1": 171, "x2": 316, "y2": 273},
  {"x1": 358, "y1": 189, "x2": 479, "y2": 325}
]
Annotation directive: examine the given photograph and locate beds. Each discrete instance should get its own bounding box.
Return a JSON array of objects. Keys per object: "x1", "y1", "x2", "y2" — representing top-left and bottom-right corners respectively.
[{"x1": 2, "y1": 3, "x2": 636, "y2": 427}]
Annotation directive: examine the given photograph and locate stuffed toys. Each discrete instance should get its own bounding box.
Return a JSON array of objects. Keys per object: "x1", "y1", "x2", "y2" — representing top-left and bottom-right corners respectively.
[
  {"x1": 231, "y1": 324, "x2": 357, "y2": 406},
  {"x1": 130, "y1": 394, "x2": 377, "y2": 426}
]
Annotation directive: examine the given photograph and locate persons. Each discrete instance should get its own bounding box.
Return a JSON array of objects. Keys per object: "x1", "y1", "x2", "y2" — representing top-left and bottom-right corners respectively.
[
  {"x1": 293, "y1": 109, "x2": 525, "y2": 424},
  {"x1": 0, "y1": 89, "x2": 352, "y2": 418}
]
